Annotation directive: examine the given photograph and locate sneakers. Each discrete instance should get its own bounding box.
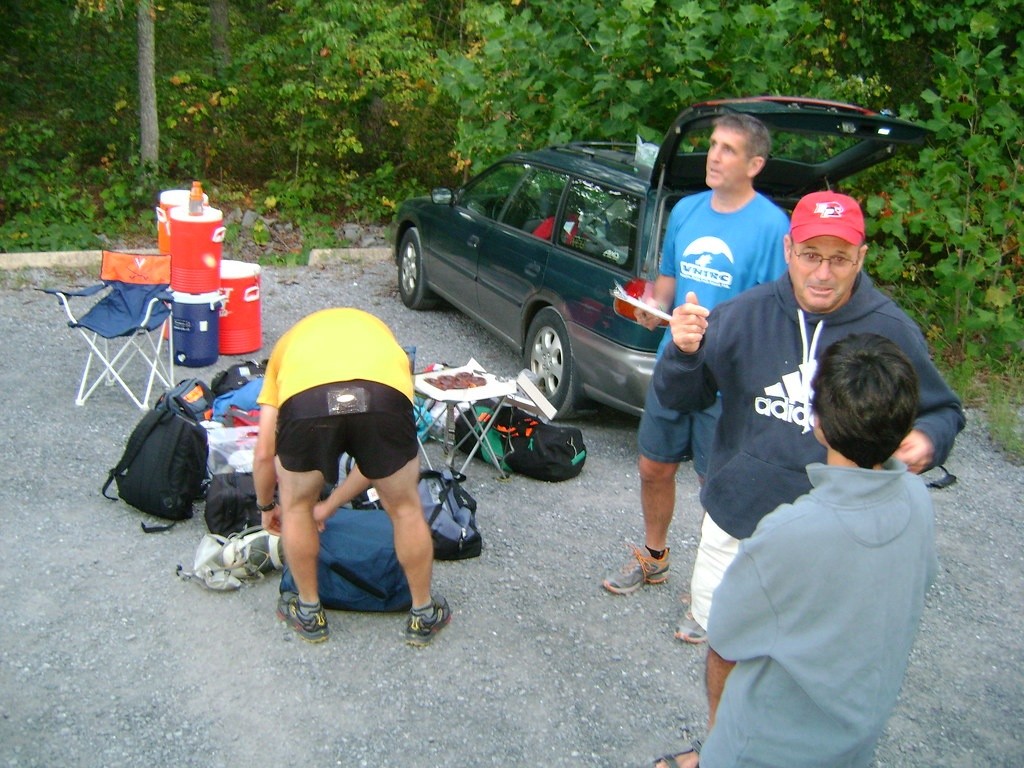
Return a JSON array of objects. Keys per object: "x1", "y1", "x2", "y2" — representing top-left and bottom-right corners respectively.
[
  {"x1": 674, "y1": 612, "x2": 709, "y2": 643},
  {"x1": 277, "y1": 592, "x2": 329, "y2": 641},
  {"x1": 407, "y1": 594, "x2": 451, "y2": 645},
  {"x1": 601, "y1": 547, "x2": 671, "y2": 593}
]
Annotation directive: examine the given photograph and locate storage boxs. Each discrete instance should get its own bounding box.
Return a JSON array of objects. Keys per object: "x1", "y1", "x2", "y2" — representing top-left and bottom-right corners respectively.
[{"x1": 415, "y1": 357, "x2": 557, "y2": 422}]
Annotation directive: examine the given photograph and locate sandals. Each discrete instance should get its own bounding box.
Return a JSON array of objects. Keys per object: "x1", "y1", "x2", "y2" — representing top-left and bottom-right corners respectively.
[{"x1": 653, "y1": 737, "x2": 705, "y2": 768}]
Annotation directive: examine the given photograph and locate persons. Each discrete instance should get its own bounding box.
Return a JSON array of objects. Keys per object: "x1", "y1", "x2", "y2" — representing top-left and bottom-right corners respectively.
[
  {"x1": 252, "y1": 308, "x2": 453, "y2": 647},
  {"x1": 695, "y1": 331, "x2": 935, "y2": 767},
  {"x1": 652, "y1": 191, "x2": 966, "y2": 768},
  {"x1": 603, "y1": 114, "x2": 790, "y2": 596}
]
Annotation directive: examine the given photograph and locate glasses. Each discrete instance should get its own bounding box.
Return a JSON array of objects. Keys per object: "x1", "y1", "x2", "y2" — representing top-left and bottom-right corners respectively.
[{"x1": 792, "y1": 243, "x2": 860, "y2": 267}]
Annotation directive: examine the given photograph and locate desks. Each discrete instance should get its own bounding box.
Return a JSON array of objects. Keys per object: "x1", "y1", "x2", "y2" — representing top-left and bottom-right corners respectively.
[{"x1": 413, "y1": 358, "x2": 558, "y2": 482}]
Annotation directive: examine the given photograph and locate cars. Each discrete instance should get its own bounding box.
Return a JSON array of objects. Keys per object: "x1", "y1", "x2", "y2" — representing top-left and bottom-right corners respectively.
[{"x1": 389, "y1": 96, "x2": 937, "y2": 423}]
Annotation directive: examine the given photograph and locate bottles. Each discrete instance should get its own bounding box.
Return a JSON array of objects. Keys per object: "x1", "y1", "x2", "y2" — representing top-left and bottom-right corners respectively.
[{"x1": 188, "y1": 182, "x2": 204, "y2": 216}]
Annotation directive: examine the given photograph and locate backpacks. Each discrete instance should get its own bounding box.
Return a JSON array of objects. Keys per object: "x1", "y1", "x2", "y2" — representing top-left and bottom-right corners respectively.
[{"x1": 103, "y1": 410, "x2": 210, "y2": 533}]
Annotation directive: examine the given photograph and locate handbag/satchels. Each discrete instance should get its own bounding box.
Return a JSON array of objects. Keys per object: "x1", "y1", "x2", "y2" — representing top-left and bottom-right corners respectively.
[
  {"x1": 415, "y1": 468, "x2": 482, "y2": 561},
  {"x1": 155, "y1": 358, "x2": 274, "y2": 429},
  {"x1": 278, "y1": 508, "x2": 412, "y2": 612},
  {"x1": 205, "y1": 472, "x2": 279, "y2": 537},
  {"x1": 414, "y1": 404, "x2": 433, "y2": 441},
  {"x1": 338, "y1": 452, "x2": 383, "y2": 509},
  {"x1": 455, "y1": 399, "x2": 587, "y2": 481}
]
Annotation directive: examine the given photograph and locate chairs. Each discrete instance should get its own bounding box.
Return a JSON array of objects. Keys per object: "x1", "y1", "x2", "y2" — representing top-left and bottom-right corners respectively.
[
  {"x1": 523, "y1": 187, "x2": 579, "y2": 246},
  {"x1": 33, "y1": 248, "x2": 176, "y2": 411}
]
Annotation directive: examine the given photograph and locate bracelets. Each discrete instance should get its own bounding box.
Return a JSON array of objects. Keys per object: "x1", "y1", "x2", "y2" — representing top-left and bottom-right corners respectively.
[{"x1": 255, "y1": 499, "x2": 277, "y2": 511}]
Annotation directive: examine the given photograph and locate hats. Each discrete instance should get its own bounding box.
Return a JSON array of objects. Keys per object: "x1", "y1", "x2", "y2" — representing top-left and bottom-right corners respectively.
[{"x1": 790, "y1": 190, "x2": 866, "y2": 246}]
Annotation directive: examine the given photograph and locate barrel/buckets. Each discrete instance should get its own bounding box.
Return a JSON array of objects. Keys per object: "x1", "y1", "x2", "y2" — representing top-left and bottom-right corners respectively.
[{"x1": 155, "y1": 189, "x2": 262, "y2": 368}]
